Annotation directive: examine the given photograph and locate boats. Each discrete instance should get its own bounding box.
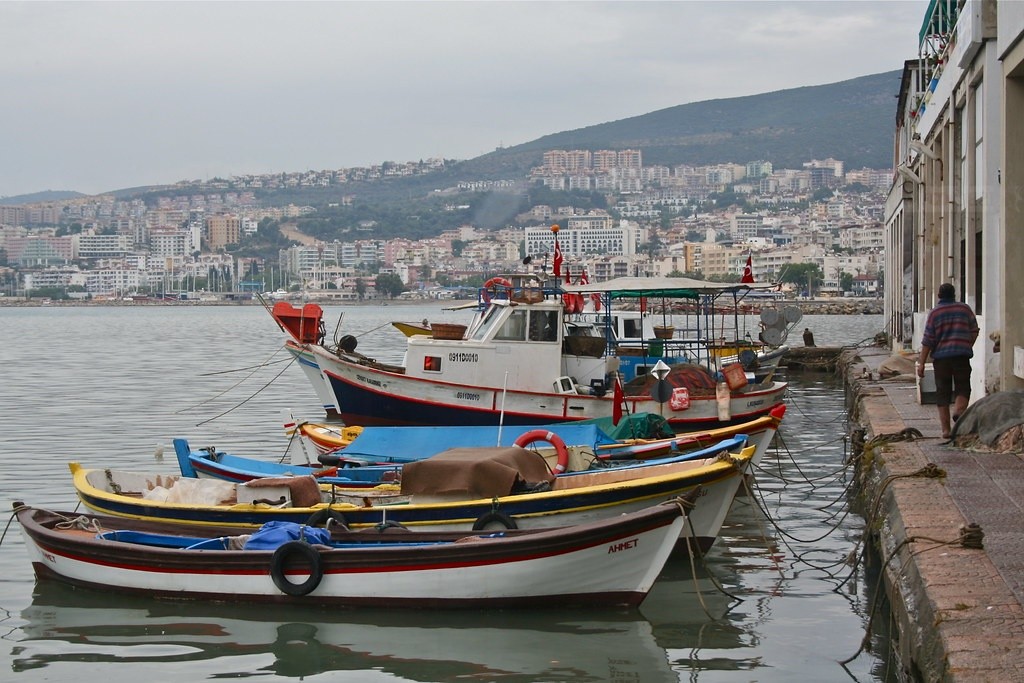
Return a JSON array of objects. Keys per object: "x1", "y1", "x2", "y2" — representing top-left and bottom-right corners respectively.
[{"x1": 10, "y1": 225, "x2": 803, "y2": 610}]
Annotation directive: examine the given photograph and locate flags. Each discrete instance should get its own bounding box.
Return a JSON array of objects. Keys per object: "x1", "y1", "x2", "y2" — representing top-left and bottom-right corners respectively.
[
  {"x1": 741, "y1": 256, "x2": 754, "y2": 283},
  {"x1": 553, "y1": 244, "x2": 563, "y2": 278},
  {"x1": 563, "y1": 271, "x2": 589, "y2": 313},
  {"x1": 612, "y1": 376, "x2": 624, "y2": 426}
]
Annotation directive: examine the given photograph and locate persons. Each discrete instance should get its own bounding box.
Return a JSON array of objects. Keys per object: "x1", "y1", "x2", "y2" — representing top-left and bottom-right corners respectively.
[{"x1": 918, "y1": 283, "x2": 980, "y2": 440}]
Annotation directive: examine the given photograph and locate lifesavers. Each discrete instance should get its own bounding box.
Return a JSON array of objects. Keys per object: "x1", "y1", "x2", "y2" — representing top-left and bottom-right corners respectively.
[
  {"x1": 306, "y1": 508, "x2": 349, "y2": 530},
  {"x1": 592, "y1": 293, "x2": 601, "y2": 311},
  {"x1": 511, "y1": 430, "x2": 569, "y2": 475},
  {"x1": 472, "y1": 510, "x2": 518, "y2": 530},
  {"x1": 269, "y1": 541, "x2": 323, "y2": 596},
  {"x1": 575, "y1": 294, "x2": 584, "y2": 313},
  {"x1": 482, "y1": 277, "x2": 515, "y2": 302},
  {"x1": 562, "y1": 283, "x2": 576, "y2": 314}
]
